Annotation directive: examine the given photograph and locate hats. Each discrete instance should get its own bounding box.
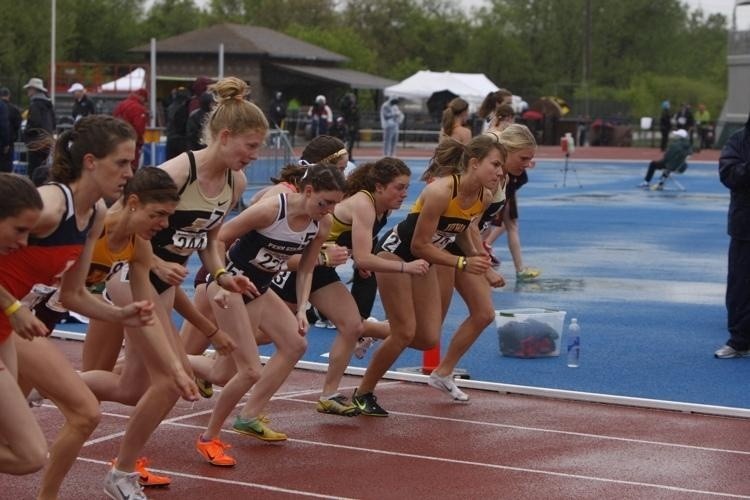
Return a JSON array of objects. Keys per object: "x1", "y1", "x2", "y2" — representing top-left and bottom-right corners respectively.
[
  {"x1": 136, "y1": 88, "x2": 150, "y2": 104},
  {"x1": 67, "y1": 82, "x2": 86, "y2": 94},
  {"x1": 22, "y1": 78, "x2": 48, "y2": 92}
]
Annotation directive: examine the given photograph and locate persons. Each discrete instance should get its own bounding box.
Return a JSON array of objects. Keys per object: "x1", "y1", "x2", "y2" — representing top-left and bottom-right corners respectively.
[
  {"x1": 437, "y1": 98, "x2": 471, "y2": 148},
  {"x1": 66, "y1": 82, "x2": 93, "y2": 117},
  {"x1": 178, "y1": 136, "x2": 348, "y2": 442},
  {"x1": 354, "y1": 124, "x2": 536, "y2": 405},
  {"x1": 480, "y1": 89, "x2": 513, "y2": 131},
  {"x1": 1, "y1": 88, "x2": 22, "y2": 171},
  {"x1": 657, "y1": 101, "x2": 711, "y2": 154},
  {"x1": 112, "y1": 89, "x2": 151, "y2": 174},
  {"x1": 196, "y1": 161, "x2": 347, "y2": 466},
  {"x1": 351, "y1": 134, "x2": 507, "y2": 417},
  {"x1": 380, "y1": 94, "x2": 404, "y2": 156},
  {"x1": 0, "y1": 172, "x2": 45, "y2": 257},
  {"x1": 163, "y1": 78, "x2": 216, "y2": 159},
  {"x1": 256, "y1": 157, "x2": 429, "y2": 419},
  {"x1": 481, "y1": 105, "x2": 539, "y2": 282},
  {"x1": 18, "y1": 166, "x2": 201, "y2": 408},
  {"x1": 22, "y1": 78, "x2": 56, "y2": 177},
  {"x1": 635, "y1": 127, "x2": 691, "y2": 191},
  {"x1": 307, "y1": 95, "x2": 333, "y2": 136},
  {"x1": 420, "y1": 185, "x2": 505, "y2": 401},
  {"x1": 0, "y1": 114, "x2": 156, "y2": 500},
  {"x1": 26, "y1": 77, "x2": 268, "y2": 500},
  {"x1": 714, "y1": 114, "x2": 750, "y2": 360}
]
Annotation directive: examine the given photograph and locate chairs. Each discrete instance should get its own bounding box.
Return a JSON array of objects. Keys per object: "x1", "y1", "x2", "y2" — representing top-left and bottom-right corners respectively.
[{"x1": 655, "y1": 150, "x2": 690, "y2": 192}]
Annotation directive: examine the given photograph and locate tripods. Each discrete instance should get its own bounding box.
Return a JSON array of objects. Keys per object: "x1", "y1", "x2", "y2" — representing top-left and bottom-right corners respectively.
[{"x1": 554, "y1": 153, "x2": 582, "y2": 188}]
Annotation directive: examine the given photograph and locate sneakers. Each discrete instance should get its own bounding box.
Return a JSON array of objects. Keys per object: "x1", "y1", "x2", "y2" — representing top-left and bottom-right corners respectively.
[
  {"x1": 352, "y1": 315, "x2": 379, "y2": 360},
  {"x1": 352, "y1": 388, "x2": 389, "y2": 418},
  {"x1": 317, "y1": 393, "x2": 361, "y2": 418},
  {"x1": 426, "y1": 369, "x2": 470, "y2": 402},
  {"x1": 515, "y1": 267, "x2": 541, "y2": 284},
  {"x1": 232, "y1": 413, "x2": 288, "y2": 443},
  {"x1": 109, "y1": 458, "x2": 171, "y2": 488},
  {"x1": 481, "y1": 239, "x2": 501, "y2": 268},
  {"x1": 194, "y1": 434, "x2": 237, "y2": 467},
  {"x1": 712, "y1": 347, "x2": 750, "y2": 359},
  {"x1": 101, "y1": 469, "x2": 147, "y2": 500},
  {"x1": 195, "y1": 351, "x2": 214, "y2": 399}
]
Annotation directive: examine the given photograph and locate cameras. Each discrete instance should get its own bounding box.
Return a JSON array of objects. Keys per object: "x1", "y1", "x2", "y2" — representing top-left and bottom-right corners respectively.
[{"x1": 313, "y1": 102, "x2": 320, "y2": 108}]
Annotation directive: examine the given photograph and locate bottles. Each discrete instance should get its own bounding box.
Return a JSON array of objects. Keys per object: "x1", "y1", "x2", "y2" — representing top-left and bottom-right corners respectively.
[{"x1": 565, "y1": 317, "x2": 580, "y2": 368}]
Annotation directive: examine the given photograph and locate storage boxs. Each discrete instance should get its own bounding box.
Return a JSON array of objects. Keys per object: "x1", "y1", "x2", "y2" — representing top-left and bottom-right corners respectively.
[{"x1": 493, "y1": 306, "x2": 567, "y2": 359}]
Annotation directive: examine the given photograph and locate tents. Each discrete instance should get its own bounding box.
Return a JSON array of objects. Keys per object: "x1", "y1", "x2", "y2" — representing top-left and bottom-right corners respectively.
[{"x1": 385, "y1": 67, "x2": 528, "y2": 117}]
